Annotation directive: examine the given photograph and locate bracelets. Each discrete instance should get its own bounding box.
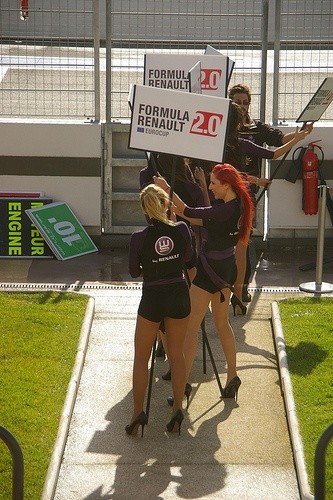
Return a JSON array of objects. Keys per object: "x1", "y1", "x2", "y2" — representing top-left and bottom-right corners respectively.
[{"x1": 164, "y1": 187, "x2": 170, "y2": 191}]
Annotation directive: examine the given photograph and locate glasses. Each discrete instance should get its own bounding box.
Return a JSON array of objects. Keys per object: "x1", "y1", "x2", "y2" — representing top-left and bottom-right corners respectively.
[{"x1": 235, "y1": 101, "x2": 250, "y2": 106}]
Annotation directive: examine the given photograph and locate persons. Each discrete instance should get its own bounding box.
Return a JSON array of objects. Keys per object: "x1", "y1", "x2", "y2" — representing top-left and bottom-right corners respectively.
[
  {"x1": 153, "y1": 163, "x2": 253, "y2": 409},
  {"x1": 125, "y1": 185, "x2": 193, "y2": 437},
  {"x1": 140, "y1": 85, "x2": 313, "y2": 381}
]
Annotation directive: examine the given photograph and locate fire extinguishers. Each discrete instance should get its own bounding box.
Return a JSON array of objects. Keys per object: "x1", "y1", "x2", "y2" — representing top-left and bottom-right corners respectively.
[{"x1": 292, "y1": 139, "x2": 325, "y2": 217}]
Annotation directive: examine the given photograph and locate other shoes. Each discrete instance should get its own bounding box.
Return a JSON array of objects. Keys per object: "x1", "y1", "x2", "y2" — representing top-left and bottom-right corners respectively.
[
  {"x1": 162, "y1": 372, "x2": 172, "y2": 380},
  {"x1": 242, "y1": 284, "x2": 252, "y2": 302}
]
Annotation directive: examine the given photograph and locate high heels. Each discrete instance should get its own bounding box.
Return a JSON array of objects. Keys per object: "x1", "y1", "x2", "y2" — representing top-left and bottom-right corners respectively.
[
  {"x1": 155, "y1": 342, "x2": 167, "y2": 360},
  {"x1": 220, "y1": 376, "x2": 242, "y2": 402},
  {"x1": 231, "y1": 294, "x2": 248, "y2": 317},
  {"x1": 167, "y1": 383, "x2": 192, "y2": 409},
  {"x1": 166, "y1": 409, "x2": 184, "y2": 436},
  {"x1": 124, "y1": 411, "x2": 147, "y2": 438}
]
